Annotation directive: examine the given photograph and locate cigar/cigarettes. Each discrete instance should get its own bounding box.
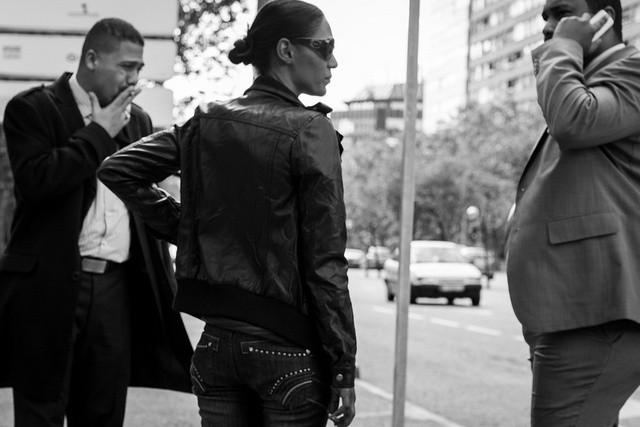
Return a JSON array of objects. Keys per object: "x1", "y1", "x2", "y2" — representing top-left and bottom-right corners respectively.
[{"x1": 129, "y1": 91, "x2": 135, "y2": 95}]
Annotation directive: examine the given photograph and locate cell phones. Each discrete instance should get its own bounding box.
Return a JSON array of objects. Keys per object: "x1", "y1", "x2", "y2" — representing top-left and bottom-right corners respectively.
[{"x1": 590, "y1": 9, "x2": 615, "y2": 42}]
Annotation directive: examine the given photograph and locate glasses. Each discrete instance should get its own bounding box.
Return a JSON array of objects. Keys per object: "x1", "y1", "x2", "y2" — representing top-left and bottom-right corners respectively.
[{"x1": 291, "y1": 36, "x2": 334, "y2": 60}]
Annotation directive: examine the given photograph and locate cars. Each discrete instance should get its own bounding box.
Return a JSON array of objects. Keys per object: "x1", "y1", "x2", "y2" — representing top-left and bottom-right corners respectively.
[
  {"x1": 382, "y1": 239, "x2": 482, "y2": 307},
  {"x1": 462, "y1": 246, "x2": 495, "y2": 278},
  {"x1": 367, "y1": 244, "x2": 389, "y2": 267}
]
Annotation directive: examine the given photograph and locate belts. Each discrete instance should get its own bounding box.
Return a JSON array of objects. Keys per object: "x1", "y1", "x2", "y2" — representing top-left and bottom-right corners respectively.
[{"x1": 73, "y1": 257, "x2": 118, "y2": 275}]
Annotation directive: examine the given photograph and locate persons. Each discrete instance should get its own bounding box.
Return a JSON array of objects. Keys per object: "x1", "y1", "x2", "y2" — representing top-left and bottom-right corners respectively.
[
  {"x1": 95, "y1": 0, "x2": 357, "y2": 427},
  {"x1": 504, "y1": 0, "x2": 639, "y2": 427},
  {"x1": 0, "y1": 19, "x2": 193, "y2": 426}
]
